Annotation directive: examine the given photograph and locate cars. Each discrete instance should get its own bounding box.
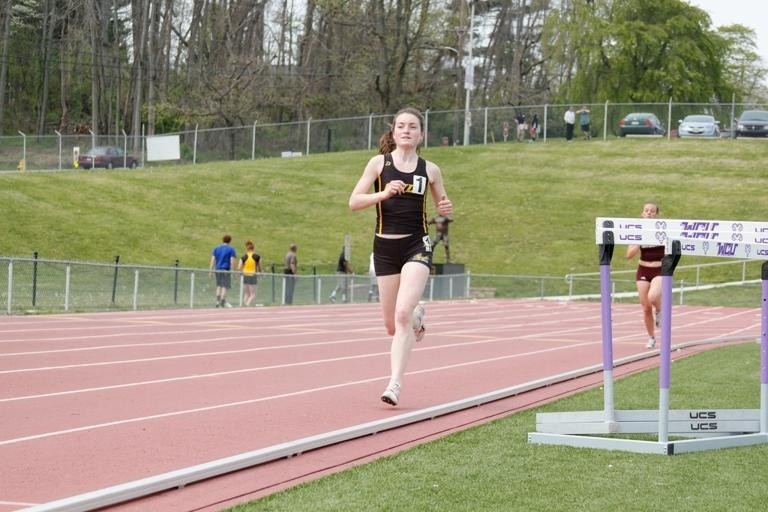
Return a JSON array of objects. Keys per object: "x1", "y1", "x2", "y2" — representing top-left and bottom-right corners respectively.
[
  {"x1": 76, "y1": 145, "x2": 141, "y2": 172},
  {"x1": 676, "y1": 113, "x2": 720, "y2": 138},
  {"x1": 731, "y1": 110, "x2": 767, "y2": 138},
  {"x1": 615, "y1": 112, "x2": 667, "y2": 138}
]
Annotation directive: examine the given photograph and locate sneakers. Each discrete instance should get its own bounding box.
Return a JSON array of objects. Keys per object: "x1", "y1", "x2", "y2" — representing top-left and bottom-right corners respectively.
[
  {"x1": 645, "y1": 336, "x2": 656, "y2": 349},
  {"x1": 328, "y1": 295, "x2": 337, "y2": 303},
  {"x1": 656, "y1": 311, "x2": 661, "y2": 328},
  {"x1": 381, "y1": 384, "x2": 400, "y2": 405},
  {"x1": 413, "y1": 304, "x2": 426, "y2": 341}
]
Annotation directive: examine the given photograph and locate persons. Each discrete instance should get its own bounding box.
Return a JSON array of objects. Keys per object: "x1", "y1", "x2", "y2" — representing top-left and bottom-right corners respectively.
[
  {"x1": 284, "y1": 242, "x2": 299, "y2": 307},
  {"x1": 563, "y1": 105, "x2": 574, "y2": 140},
  {"x1": 237, "y1": 241, "x2": 265, "y2": 307},
  {"x1": 348, "y1": 106, "x2": 454, "y2": 409},
  {"x1": 625, "y1": 202, "x2": 665, "y2": 351},
  {"x1": 514, "y1": 111, "x2": 525, "y2": 141},
  {"x1": 330, "y1": 245, "x2": 353, "y2": 304},
  {"x1": 207, "y1": 234, "x2": 237, "y2": 310},
  {"x1": 366, "y1": 250, "x2": 380, "y2": 305},
  {"x1": 503, "y1": 121, "x2": 509, "y2": 141},
  {"x1": 575, "y1": 106, "x2": 595, "y2": 140},
  {"x1": 529, "y1": 112, "x2": 539, "y2": 142}
]
